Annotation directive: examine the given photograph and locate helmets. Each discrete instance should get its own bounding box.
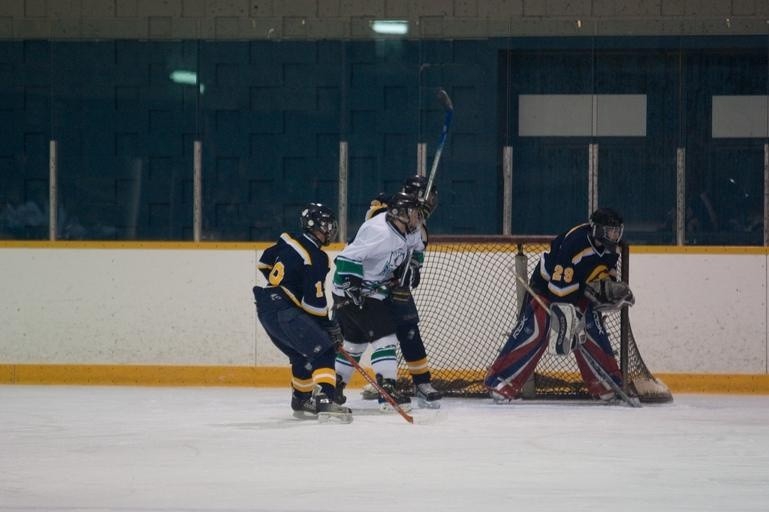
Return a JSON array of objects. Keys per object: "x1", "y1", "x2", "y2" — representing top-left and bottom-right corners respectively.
[
  {"x1": 299, "y1": 202, "x2": 334, "y2": 234},
  {"x1": 590, "y1": 208, "x2": 623, "y2": 239},
  {"x1": 386, "y1": 175, "x2": 439, "y2": 224}
]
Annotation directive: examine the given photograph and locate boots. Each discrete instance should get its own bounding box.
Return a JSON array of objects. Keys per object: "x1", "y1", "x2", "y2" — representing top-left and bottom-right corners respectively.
[
  {"x1": 417, "y1": 383, "x2": 442, "y2": 402},
  {"x1": 291, "y1": 373, "x2": 352, "y2": 413},
  {"x1": 375, "y1": 374, "x2": 411, "y2": 404}
]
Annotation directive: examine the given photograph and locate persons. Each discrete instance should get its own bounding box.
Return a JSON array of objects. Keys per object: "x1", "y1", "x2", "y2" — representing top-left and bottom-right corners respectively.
[
  {"x1": 484, "y1": 206, "x2": 634, "y2": 400},
  {"x1": 330, "y1": 173, "x2": 442, "y2": 401},
  {"x1": 253, "y1": 203, "x2": 346, "y2": 414}
]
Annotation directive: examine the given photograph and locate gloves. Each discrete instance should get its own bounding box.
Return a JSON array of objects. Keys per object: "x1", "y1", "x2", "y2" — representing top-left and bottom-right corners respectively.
[
  {"x1": 549, "y1": 303, "x2": 586, "y2": 354},
  {"x1": 585, "y1": 281, "x2": 634, "y2": 312},
  {"x1": 327, "y1": 320, "x2": 343, "y2": 349},
  {"x1": 411, "y1": 268, "x2": 420, "y2": 288}
]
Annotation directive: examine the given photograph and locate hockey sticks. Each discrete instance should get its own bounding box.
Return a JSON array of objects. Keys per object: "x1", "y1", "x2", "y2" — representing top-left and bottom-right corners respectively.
[
  {"x1": 336, "y1": 343, "x2": 433, "y2": 425},
  {"x1": 398, "y1": 88, "x2": 453, "y2": 287},
  {"x1": 508, "y1": 263, "x2": 641, "y2": 407}
]
